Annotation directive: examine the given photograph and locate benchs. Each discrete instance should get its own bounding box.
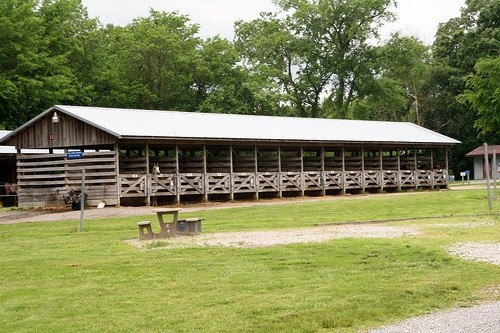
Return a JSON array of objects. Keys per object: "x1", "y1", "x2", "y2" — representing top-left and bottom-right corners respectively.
[
  {"x1": 137, "y1": 221, "x2": 154, "y2": 240},
  {"x1": 184, "y1": 217, "x2": 200, "y2": 235},
  {"x1": 173, "y1": 219, "x2": 203, "y2": 232}
]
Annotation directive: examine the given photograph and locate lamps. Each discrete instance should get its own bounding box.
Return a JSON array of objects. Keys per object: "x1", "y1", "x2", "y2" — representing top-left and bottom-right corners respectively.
[{"x1": 52, "y1": 111, "x2": 59, "y2": 123}]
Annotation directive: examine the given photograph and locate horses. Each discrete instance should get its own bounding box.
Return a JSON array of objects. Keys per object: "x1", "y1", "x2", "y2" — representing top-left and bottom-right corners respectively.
[
  {"x1": 149, "y1": 163, "x2": 161, "y2": 207},
  {"x1": 0, "y1": 182, "x2": 17, "y2": 207}
]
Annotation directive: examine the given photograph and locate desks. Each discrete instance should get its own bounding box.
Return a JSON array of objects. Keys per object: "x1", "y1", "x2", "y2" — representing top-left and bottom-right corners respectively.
[{"x1": 151, "y1": 208, "x2": 183, "y2": 237}]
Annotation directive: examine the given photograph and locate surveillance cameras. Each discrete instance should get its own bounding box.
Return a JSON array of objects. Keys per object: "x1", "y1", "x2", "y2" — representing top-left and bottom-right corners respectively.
[{"x1": 52, "y1": 112, "x2": 59, "y2": 124}]
